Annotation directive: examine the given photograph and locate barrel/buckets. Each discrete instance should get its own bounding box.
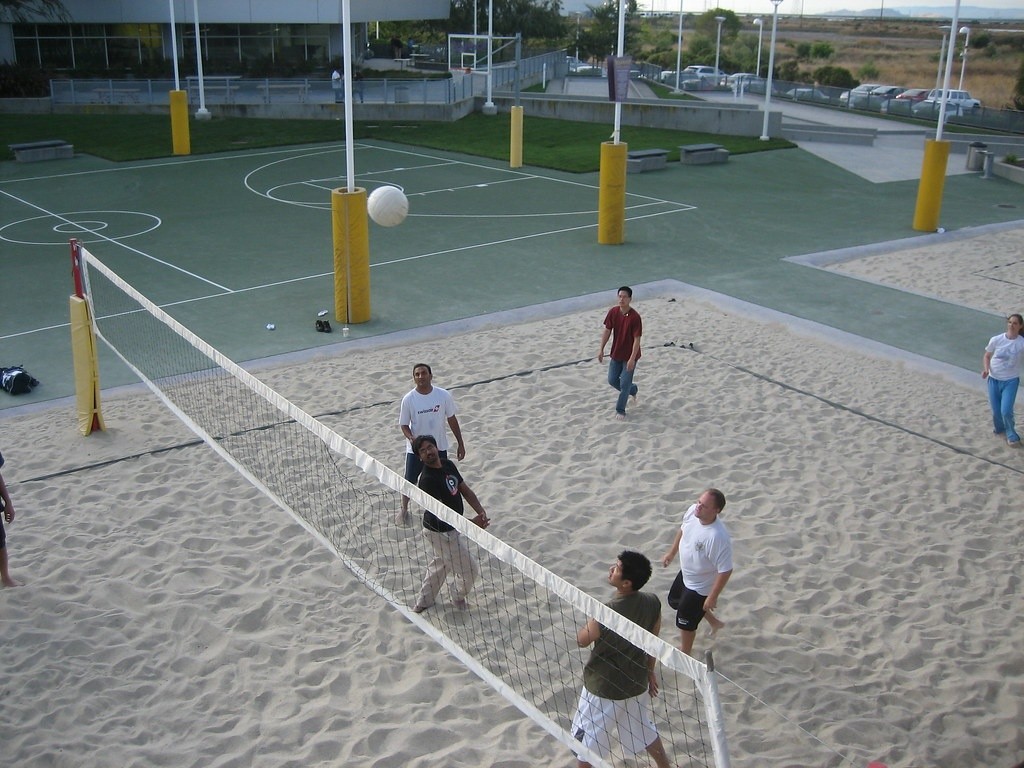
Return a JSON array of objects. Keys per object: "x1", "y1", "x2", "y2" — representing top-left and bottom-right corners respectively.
[{"x1": 965, "y1": 141, "x2": 990, "y2": 170}]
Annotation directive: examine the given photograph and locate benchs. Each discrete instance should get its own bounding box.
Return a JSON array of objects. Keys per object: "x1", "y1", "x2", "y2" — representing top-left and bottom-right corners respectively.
[
  {"x1": 8, "y1": 141, "x2": 74, "y2": 163},
  {"x1": 627, "y1": 149, "x2": 669, "y2": 174},
  {"x1": 678, "y1": 143, "x2": 729, "y2": 165}
]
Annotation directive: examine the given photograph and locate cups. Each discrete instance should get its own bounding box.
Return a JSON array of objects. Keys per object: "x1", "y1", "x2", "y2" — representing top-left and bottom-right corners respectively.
[{"x1": 342, "y1": 328, "x2": 349, "y2": 337}]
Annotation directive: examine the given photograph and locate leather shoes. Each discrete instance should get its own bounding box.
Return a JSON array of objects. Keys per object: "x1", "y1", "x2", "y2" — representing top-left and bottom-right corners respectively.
[
  {"x1": 316, "y1": 320, "x2": 324, "y2": 331},
  {"x1": 323, "y1": 321, "x2": 331, "y2": 333}
]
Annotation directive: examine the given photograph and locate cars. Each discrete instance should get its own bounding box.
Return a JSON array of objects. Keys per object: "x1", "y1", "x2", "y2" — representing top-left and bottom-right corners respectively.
[
  {"x1": 720, "y1": 73, "x2": 767, "y2": 92},
  {"x1": 869, "y1": 86, "x2": 909, "y2": 98},
  {"x1": 841, "y1": 84, "x2": 963, "y2": 122},
  {"x1": 567, "y1": 56, "x2": 713, "y2": 90},
  {"x1": 786, "y1": 88, "x2": 830, "y2": 100},
  {"x1": 683, "y1": 65, "x2": 729, "y2": 85},
  {"x1": 896, "y1": 88, "x2": 930, "y2": 101}
]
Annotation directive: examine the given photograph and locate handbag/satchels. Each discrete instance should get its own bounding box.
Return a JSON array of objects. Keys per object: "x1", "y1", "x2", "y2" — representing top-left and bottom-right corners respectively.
[{"x1": 1, "y1": 366, "x2": 39, "y2": 393}]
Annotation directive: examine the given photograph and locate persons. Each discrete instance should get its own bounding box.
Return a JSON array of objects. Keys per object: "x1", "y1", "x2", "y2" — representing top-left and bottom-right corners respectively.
[
  {"x1": 570, "y1": 550, "x2": 671, "y2": 768},
  {"x1": 982, "y1": 314, "x2": 1024, "y2": 446},
  {"x1": 332, "y1": 67, "x2": 343, "y2": 103},
  {"x1": 663, "y1": 489, "x2": 733, "y2": 655},
  {"x1": 411, "y1": 434, "x2": 490, "y2": 614},
  {"x1": 0, "y1": 452, "x2": 26, "y2": 589},
  {"x1": 352, "y1": 72, "x2": 364, "y2": 103},
  {"x1": 390, "y1": 34, "x2": 403, "y2": 58},
  {"x1": 407, "y1": 38, "x2": 415, "y2": 59},
  {"x1": 599, "y1": 286, "x2": 642, "y2": 419},
  {"x1": 395, "y1": 363, "x2": 465, "y2": 524}
]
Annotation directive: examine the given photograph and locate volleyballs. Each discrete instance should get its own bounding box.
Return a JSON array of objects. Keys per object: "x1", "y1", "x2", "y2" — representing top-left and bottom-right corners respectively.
[{"x1": 367, "y1": 185, "x2": 408, "y2": 228}]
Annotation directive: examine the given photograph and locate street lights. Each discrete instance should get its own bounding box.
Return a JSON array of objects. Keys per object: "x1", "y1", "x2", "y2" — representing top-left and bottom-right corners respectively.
[
  {"x1": 759, "y1": 0, "x2": 783, "y2": 140},
  {"x1": 575, "y1": 12, "x2": 581, "y2": 72},
  {"x1": 930, "y1": 26, "x2": 951, "y2": 121},
  {"x1": 714, "y1": 16, "x2": 726, "y2": 87}
]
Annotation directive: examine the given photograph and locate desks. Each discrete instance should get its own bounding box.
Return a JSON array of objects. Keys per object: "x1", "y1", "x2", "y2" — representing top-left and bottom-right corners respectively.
[
  {"x1": 185, "y1": 86, "x2": 240, "y2": 104},
  {"x1": 93, "y1": 88, "x2": 140, "y2": 103},
  {"x1": 257, "y1": 84, "x2": 311, "y2": 103},
  {"x1": 395, "y1": 59, "x2": 411, "y2": 70}
]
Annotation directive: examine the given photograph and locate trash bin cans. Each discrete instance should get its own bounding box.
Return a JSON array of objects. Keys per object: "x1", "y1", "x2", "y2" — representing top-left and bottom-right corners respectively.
[{"x1": 965, "y1": 142, "x2": 988, "y2": 172}]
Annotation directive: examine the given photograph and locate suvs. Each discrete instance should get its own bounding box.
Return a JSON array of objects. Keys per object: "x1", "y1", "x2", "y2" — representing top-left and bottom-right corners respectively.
[{"x1": 927, "y1": 89, "x2": 981, "y2": 114}]
